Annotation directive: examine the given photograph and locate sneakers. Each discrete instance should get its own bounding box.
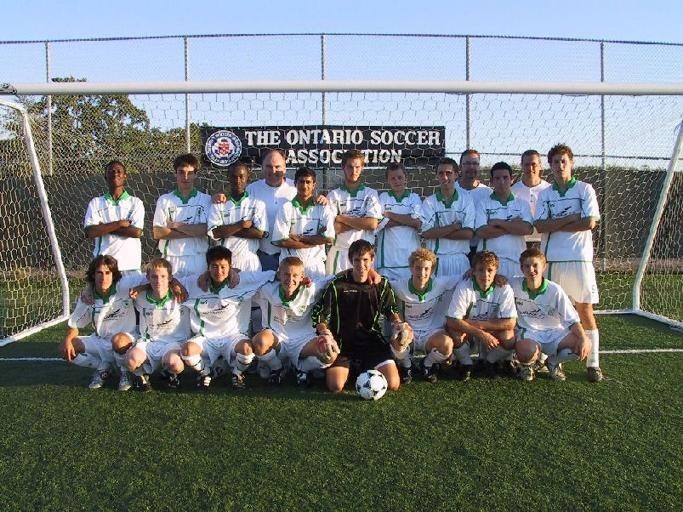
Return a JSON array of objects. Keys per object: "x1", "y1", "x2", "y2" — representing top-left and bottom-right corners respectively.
[
  {"x1": 459, "y1": 364, "x2": 474, "y2": 382},
  {"x1": 399, "y1": 365, "x2": 414, "y2": 386},
  {"x1": 197, "y1": 367, "x2": 217, "y2": 392},
  {"x1": 133, "y1": 373, "x2": 154, "y2": 393},
  {"x1": 519, "y1": 364, "x2": 536, "y2": 381},
  {"x1": 231, "y1": 371, "x2": 248, "y2": 392},
  {"x1": 586, "y1": 366, "x2": 603, "y2": 384},
  {"x1": 167, "y1": 369, "x2": 181, "y2": 389},
  {"x1": 267, "y1": 365, "x2": 287, "y2": 387},
  {"x1": 420, "y1": 358, "x2": 439, "y2": 383},
  {"x1": 295, "y1": 367, "x2": 309, "y2": 388},
  {"x1": 89, "y1": 369, "x2": 114, "y2": 389},
  {"x1": 118, "y1": 370, "x2": 135, "y2": 392},
  {"x1": 545, "y1": 358, "x2": 566, "y2": 382}
]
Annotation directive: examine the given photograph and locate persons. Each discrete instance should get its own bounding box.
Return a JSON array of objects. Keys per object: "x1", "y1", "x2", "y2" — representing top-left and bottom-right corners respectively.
[
  {"x1": 128, "y1": 245, "x2": 313, "y2": 392},
  {"x1": 271, "y1": 167, "x2": 335, "y2": 376},
  {"x1": 388, "y1": 247, "x2": 509, "y2": 385},
  {"x1": 322, "y1": 150, "x2": 383, "y2": 368},
  {"x1": 310, "y1": 239, "x2": 414, "y2": 393},
  {"x1": 206, "y1": 161, "x2": 269, "y2": 375},
  {"x1": 152, "y1": 154, "x2": 212, "y2": 279},
  {"x1": 376, "y1": 161, "x2": 427, "y2": 376},
  {"x1": 81, "y1": 258, "x2": 240, "y2": 395},
  {"x1": 58, "y1": 254, "x2": 189, "y2": 392},
  {"x1": 510, "y1": 150, "x2": 551, "y2": 371},
  {"x1": 533, "y1": 143, "x2": 603, "y2": 383},
  {"x1": 84, "y1": 161, "x2": 146, "y2": 325},
  {"x1": 445, "y1": 250, "x2": 517, "y2": 382},
  {"x1": 476, "y1": 162, "x2": 534, "y2": 369},
  {"x1": 213, "y1": 149, "x2": 328, "y2": 376},
  {"x1": 493, "y1": 248, "x2": 589, "y2": 382},
  {"x1": 456, "y1": 149, "x2": 495, "y2": 358},
  {"x1": 419, "y1": 157, "x2": 476, "y2": 369}
]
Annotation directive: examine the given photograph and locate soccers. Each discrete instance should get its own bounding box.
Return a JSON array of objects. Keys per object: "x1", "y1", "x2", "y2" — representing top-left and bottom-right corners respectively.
[{"x1": 355, "y1": 370, "x2": 387, "y2": 400}]
